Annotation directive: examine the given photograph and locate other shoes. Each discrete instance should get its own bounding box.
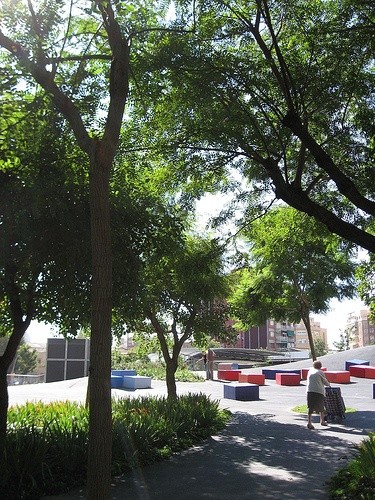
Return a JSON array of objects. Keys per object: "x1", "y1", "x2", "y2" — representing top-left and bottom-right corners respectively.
[
  {"x1": 321, "y1": 420, "x2": 327, "y2": 426},
  {"x1": 307, "y1": 424, "x2": 315, "y2": 429}
]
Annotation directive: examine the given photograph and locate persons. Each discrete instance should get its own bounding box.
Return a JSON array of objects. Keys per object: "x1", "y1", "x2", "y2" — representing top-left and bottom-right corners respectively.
[
  {"x1": 307, "y1": 361, "x2": 331, "y2": 428},
  {"x1": 205, "y1": 348, "x2": 213, "y2": 379}
]
